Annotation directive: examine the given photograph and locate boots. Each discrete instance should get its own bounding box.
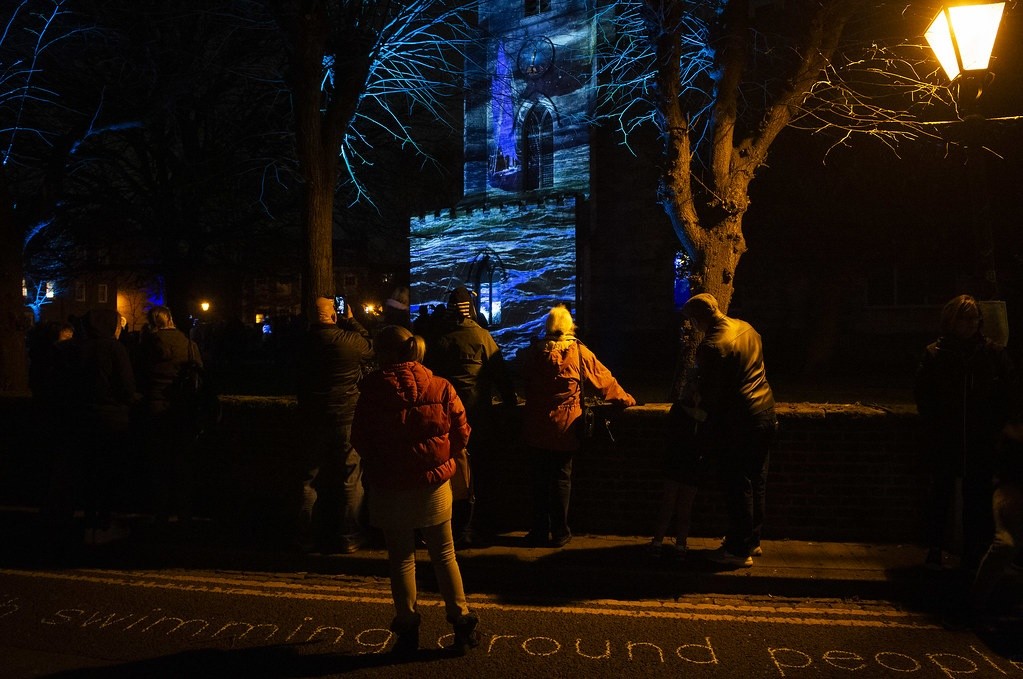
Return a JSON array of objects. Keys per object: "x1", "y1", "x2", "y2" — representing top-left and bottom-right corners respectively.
[
  {"x1": 392, "y1": 614, "x2": 421, "y2": 654},
  {"x1": 447, "y1": 611, "x2": 480, "y2": 654}
]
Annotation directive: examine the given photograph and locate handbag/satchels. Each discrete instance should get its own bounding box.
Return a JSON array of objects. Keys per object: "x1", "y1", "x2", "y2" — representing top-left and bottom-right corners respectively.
[
  {"x1": 181, "y1": 336, "x2": 204, "y2": 395},
  {"x1": 582, "y1": 410, "x2": 595, "y2": 441}
]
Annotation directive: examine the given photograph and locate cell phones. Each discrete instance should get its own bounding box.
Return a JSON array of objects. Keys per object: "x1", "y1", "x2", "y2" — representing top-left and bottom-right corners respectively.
[{"x1": 334, "y1": 295, "x2": 347, "y2": 316}]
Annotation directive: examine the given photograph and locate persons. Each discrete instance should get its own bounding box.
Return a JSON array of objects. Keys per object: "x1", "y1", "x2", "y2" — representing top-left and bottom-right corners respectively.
[
  {"x1": 289, "y1": 288, "x2": 647, "y2": 657},
  {"x1": 29, "y1": 306, "x2": 223, "y2": 546},
  {"x1": 914, "y1": 293, "x2": 1022, "y2": 575},
  {"x1": 651, "y1": 292, "x2": 781, "y2": 569}
]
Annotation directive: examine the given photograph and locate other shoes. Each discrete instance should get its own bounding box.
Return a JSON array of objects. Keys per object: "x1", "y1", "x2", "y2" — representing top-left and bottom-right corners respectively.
[
  {"x1": 925, "y1": 548, "x2": 942, "y2": 569},
  {"x1": 705, "y1": 546, "x2": 753, "y2": 567},
  {"x1": 723, "y1": 535, "x2": 762, "y2": 557},
  {"x1": 341, "y1": 535, "x2": 370, "y2": 553},
  {"x1": 673, "y1": 545, "x2": 687, "y2": 563},
  {"x1": 529, "y1": 530, "x2": 552, "y2": 545},
  {"x1": 1010, "y1": 552, "x2": 1023, "y2": 570},
  {"x1": 554, "y1": 527, "x2": 571, "y2": 546},
  {"x1": 648, "y1": 542, "x2": 664, "y2": 566},
  {"x1": 85, "y1": 528, "x2": 128, "y2": 546}
]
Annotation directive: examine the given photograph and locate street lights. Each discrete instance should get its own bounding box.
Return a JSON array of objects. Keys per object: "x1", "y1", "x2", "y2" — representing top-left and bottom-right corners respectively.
[{"x1": 922, "y1": 0, "x2": 1007, "y2": 298}]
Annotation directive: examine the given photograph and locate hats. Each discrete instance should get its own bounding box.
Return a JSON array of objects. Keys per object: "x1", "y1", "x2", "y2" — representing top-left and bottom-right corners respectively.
[{"x1": 682, "y1": 293, "x2": 718, "y2": 319}]
